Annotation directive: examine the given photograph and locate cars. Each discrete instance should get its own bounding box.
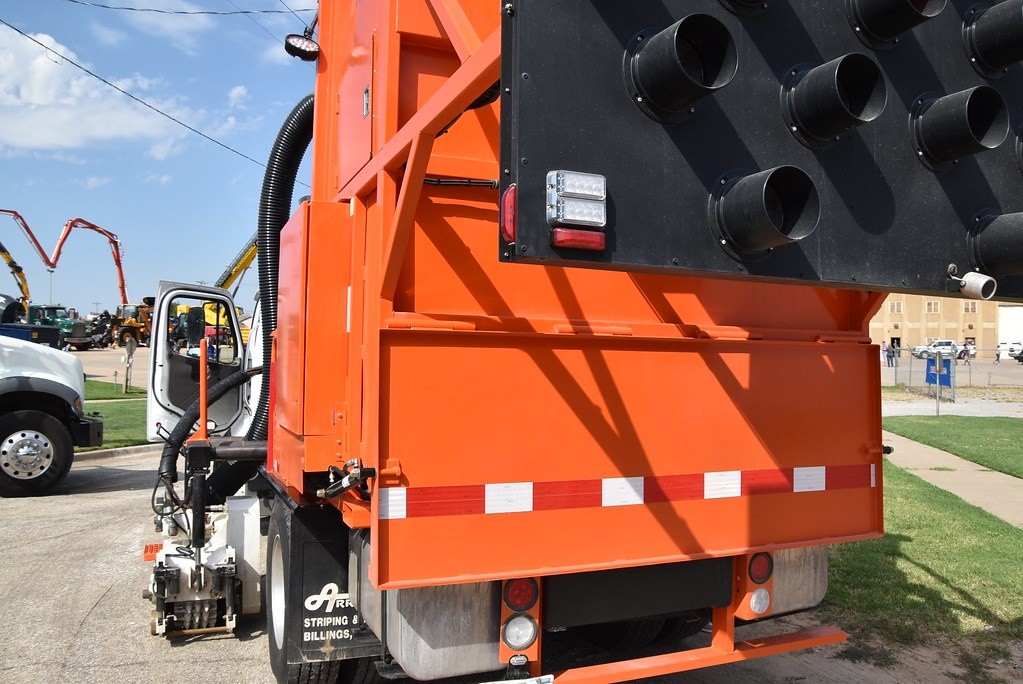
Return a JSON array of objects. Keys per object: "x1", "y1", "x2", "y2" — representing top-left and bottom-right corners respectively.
[{"x1": 0, "y1": 333, "x2": 104, "y2": 499}]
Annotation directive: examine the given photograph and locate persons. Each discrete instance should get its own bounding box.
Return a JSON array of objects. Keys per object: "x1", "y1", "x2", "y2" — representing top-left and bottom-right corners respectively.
[
  {"x1": 207, "y1": 341, "x2": 215, "y2": 360},
  {"x1": 886, "y1": 344, "x2": 893, "y2": 367},
  {"x1": 894, "y1": 343, "x2": 900, "y2": 366},
  {"x1": 952, "y1": 344, "x2": 959, "y2": 365},
  {"x1": 114, "y1": 328, "x2": 119, "y2": 343},
  {"x1": 962, "y1": 344, "x2": 970, "y2": 365},
  {"x1": 192, "y1": 317, "x2": 203, "y2": 343},
  {"x1": 993, "y1": 345, "x2": 1001, "y2": 362},
  {"x1": 882, "y1": 341, "x2": 887, "y2": 364}
]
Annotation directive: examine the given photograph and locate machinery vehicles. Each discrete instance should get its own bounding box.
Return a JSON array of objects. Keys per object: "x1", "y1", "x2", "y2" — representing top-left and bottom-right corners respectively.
[
  {"x1": 138, "y1": 1, "x2": 1023, "y2": 684},
  {"x1": 0, "y1": 208, "x2": 259, "y2": 347}
]
